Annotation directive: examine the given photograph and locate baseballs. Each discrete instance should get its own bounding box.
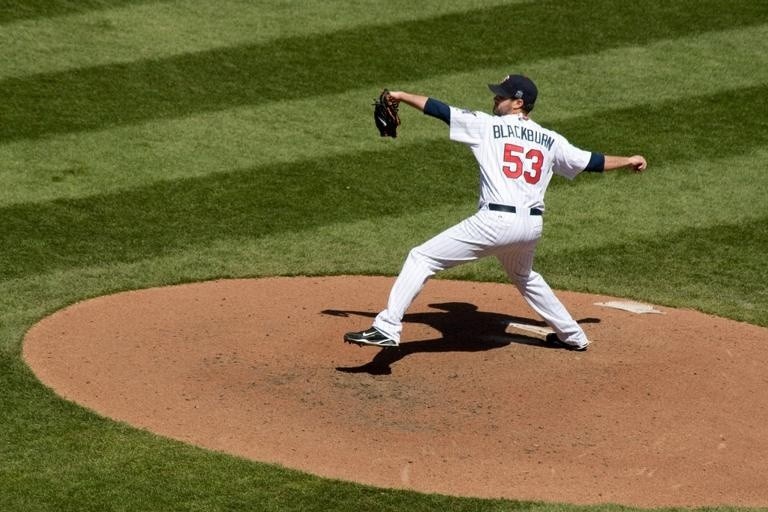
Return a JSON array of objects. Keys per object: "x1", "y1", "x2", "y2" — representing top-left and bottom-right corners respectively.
[{"x1": 630, "y1": 164, "x2": 642, "y2": 172}]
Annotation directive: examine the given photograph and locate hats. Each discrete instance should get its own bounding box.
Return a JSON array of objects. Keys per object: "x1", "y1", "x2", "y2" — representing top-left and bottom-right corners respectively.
[{"x1": 487, "y1": 72, "x2": 539, "y2": 113}]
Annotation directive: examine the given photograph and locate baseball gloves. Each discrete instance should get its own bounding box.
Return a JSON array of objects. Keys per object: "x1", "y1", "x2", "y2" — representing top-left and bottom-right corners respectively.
[{"x1": 374, "y1": 88, "x2": 400, "y2": 138}]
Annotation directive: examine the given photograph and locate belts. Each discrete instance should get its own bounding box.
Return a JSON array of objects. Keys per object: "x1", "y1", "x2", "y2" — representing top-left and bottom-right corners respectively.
[{"x1": 479, "y1": 202, "x2": 544, "y2": 217}]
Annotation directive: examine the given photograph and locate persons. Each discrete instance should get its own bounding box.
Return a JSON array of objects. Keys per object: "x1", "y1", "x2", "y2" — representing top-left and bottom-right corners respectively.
[{"x1": 342, "y1": 73, "x2": 648, "y2": 354}]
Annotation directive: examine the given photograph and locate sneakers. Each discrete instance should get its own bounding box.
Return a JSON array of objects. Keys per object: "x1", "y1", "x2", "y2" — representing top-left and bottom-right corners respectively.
[
  {"x1": 342, "y1": 325, "x2": 400, "y2": 350},
  {"x1": 546, "y1": 332, "x2": 587, "y2": 352}
]
